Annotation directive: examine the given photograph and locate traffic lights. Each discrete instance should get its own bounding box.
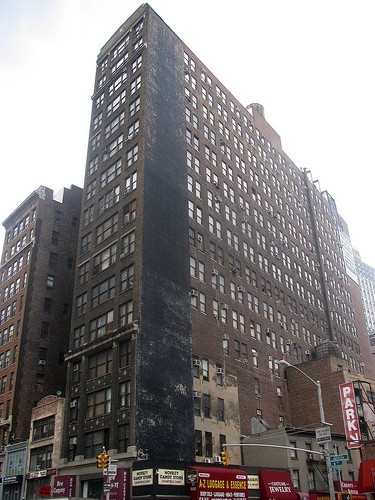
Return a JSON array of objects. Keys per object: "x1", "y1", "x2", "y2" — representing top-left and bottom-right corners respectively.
[
  {"x1": 220, "y1": 450, "x2": 228, "y2": 468},
  {"x1": 103, "y1": 454, "x2": 110, "y2": 468},
  {"x1": 96, "y1": 454, "x2": 103, "y2": 469}
]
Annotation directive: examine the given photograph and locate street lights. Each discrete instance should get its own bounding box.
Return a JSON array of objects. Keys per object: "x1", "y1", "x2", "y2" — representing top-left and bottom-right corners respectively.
[{"x1": 276, "y1": 359, "x2": 336, "y2": 500}]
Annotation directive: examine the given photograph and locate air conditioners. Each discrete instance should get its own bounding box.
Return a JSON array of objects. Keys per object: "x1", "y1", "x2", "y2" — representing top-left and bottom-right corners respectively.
[
  {"x1": 214, "y1": 183, "x2": 220, "y2": 188},
  {"x1": 305, "y1": 349, "x2": 311, "y2": 355},
  {"x1": 282, "y1": 240, "x2": 286, "y2": 244},
  {"x1": 275, "y1": 255, "x2": 281, "y2": 260},
  {"x1": 69, "y1": 437, "x2": 77, "y2": 445},
  {"x1": 39, "y1": 360, "x2": 46, "y2": 365},
  {"x1": 231, "y1": 267, "x2": 237, "y2": 273},
  {"x1": 286, "y1": 339, "x2": 292, "y2": 345},
  {"x1": 223, "y1": 334, "x2": 229, "y2": 340},
  {"x1": 123, "y1": 188, "x2": 130, "y2": 195},
  {"x1": 277, "y1": 392, "x2": 282, "y2": 397},
  {"x1": 239, "y1": 286, "x2": 245, "y2": 292},
  {"x1": 194, "y1": 391, "x2": 201, "y2": 398},
  {"x1": 211, "y1": 269, "x2": 219, "y2": 276},
  {"x1": 221, "y1": 138, "x2": 226, "y2": 145},
  {"x1": 214, "y1": 196, "x2": 223, "y2": 203},
  {"x1": 103, "y1": 204, "x2": 108, "y2": 210},
  {"x1": 243, "y1": 358, "x2": 248, "y2": 364},
  {"x1": 191, "y1": 290, "x2": 198, "y2": 297},
  {"x1": 222, "y1": 304, "x2": 229, "y2": 309},
  {"x1": 193, "y1": 359, "x2": 201, "y2": 367},
  {"x1": 34, "y1": 465, "x2": 41, "y2": 471},
  {"x1": 74, "y1": 365, "x2": 78, "y2": 371},
  {"x1": 217, "y1": 368, "x2": 225, "y2": 375},
  {"x1": 315, "y1": 262, "x2": 321, "y2": 267},
  {"x1": 241, "y1": 217, "x2": 248, "y2": 223},
  {"x1": 266, "y1": 328, "x2": 272, "y2": 336}
]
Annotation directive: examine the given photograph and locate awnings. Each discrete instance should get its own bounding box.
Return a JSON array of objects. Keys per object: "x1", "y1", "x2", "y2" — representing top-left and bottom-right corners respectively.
[
  {"x1": 258, "y1": 469, "x2": 298, "y2": 500},
  {"x1": 295, "y1": 492, "x2": 310, "y2": 500}
]
